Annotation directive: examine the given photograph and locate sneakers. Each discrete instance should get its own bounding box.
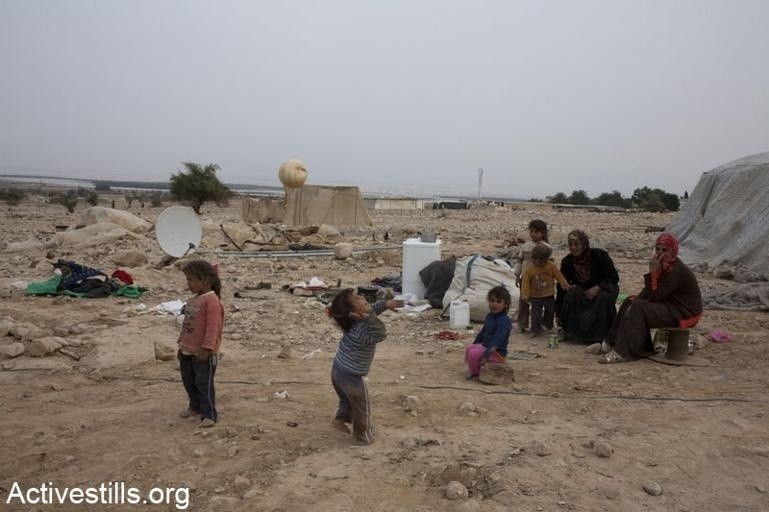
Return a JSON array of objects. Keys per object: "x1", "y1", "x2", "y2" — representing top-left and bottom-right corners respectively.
[{"x1": 598, "y1": 342, "x2": 628, "y2": 364}]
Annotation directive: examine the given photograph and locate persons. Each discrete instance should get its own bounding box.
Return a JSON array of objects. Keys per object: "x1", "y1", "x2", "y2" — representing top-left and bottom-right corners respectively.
[
  {"x1": 177, "y1": 260, "x2": 225, "y2": 428},
  {"x1": 329, "y1": 287, "x2": 405, "y2": 445},
  {"x1": 520, "y1": 240, "x2": 577, "y2": 338},
  {"x1": 597, "y1": 232, "x2": 703, "y2": 364},
  {"x1": 513, "y1": 217, "x2": 556, "y2": 335},
  {"x1": 554, "y1": 230, "x2": 620, "y2": 342},
  {"x1": 465, "y1": 283, "x2": 514, "y2": 384}
]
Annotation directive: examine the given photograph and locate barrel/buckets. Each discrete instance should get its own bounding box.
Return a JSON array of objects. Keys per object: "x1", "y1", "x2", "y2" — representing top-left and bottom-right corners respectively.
[{"x1": 449, "y1": 299, "x2": 471, "y2": 328}]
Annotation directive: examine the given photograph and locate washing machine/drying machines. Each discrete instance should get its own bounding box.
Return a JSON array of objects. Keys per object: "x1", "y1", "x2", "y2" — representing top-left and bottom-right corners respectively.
[{"x1": 401, "y1": 236, "x2": 442, "y2": 300}]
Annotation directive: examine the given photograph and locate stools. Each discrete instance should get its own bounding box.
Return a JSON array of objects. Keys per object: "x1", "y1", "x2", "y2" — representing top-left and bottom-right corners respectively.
[{"x1": 647, "y1": 327, "x2": 712, "y2": 368}]
